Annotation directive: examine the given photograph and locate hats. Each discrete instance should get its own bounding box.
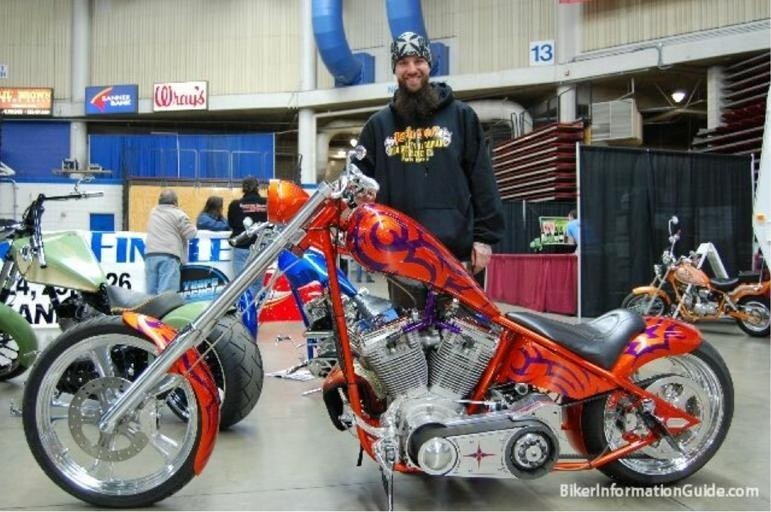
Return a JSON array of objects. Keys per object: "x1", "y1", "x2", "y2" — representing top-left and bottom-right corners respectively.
[{"x1": 390, "y1": 31, "x2": 434, "y2": 73}]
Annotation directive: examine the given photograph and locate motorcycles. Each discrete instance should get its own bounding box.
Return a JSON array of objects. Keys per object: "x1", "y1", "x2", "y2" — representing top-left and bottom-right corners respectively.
[{"x1": 0, "y1": 145, "x2": 771, "y2": 511}]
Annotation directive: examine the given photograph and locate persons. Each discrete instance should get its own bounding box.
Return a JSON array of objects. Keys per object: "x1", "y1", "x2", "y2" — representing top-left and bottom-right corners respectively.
[
  {"x1": 227, "y1": 175, "x2": 268, "y2": 299},
  {"x1": 348, "y1": 31, "x2": 506, "y2": 312},
  {"x1": 143, "y1": 188, "x2": 198, "y2": 296},
  {"x1": 196, "y1": 196, "x2": 230, "y2": 230},
  {"x1": 563, "y1": 208, "x2": 578, "y2": 254}
]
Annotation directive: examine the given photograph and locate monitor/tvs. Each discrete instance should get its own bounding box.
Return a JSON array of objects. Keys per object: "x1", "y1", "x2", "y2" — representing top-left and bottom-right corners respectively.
[{"x1": 538, "y1": 215, "x2": 576, "y2": 253}]
